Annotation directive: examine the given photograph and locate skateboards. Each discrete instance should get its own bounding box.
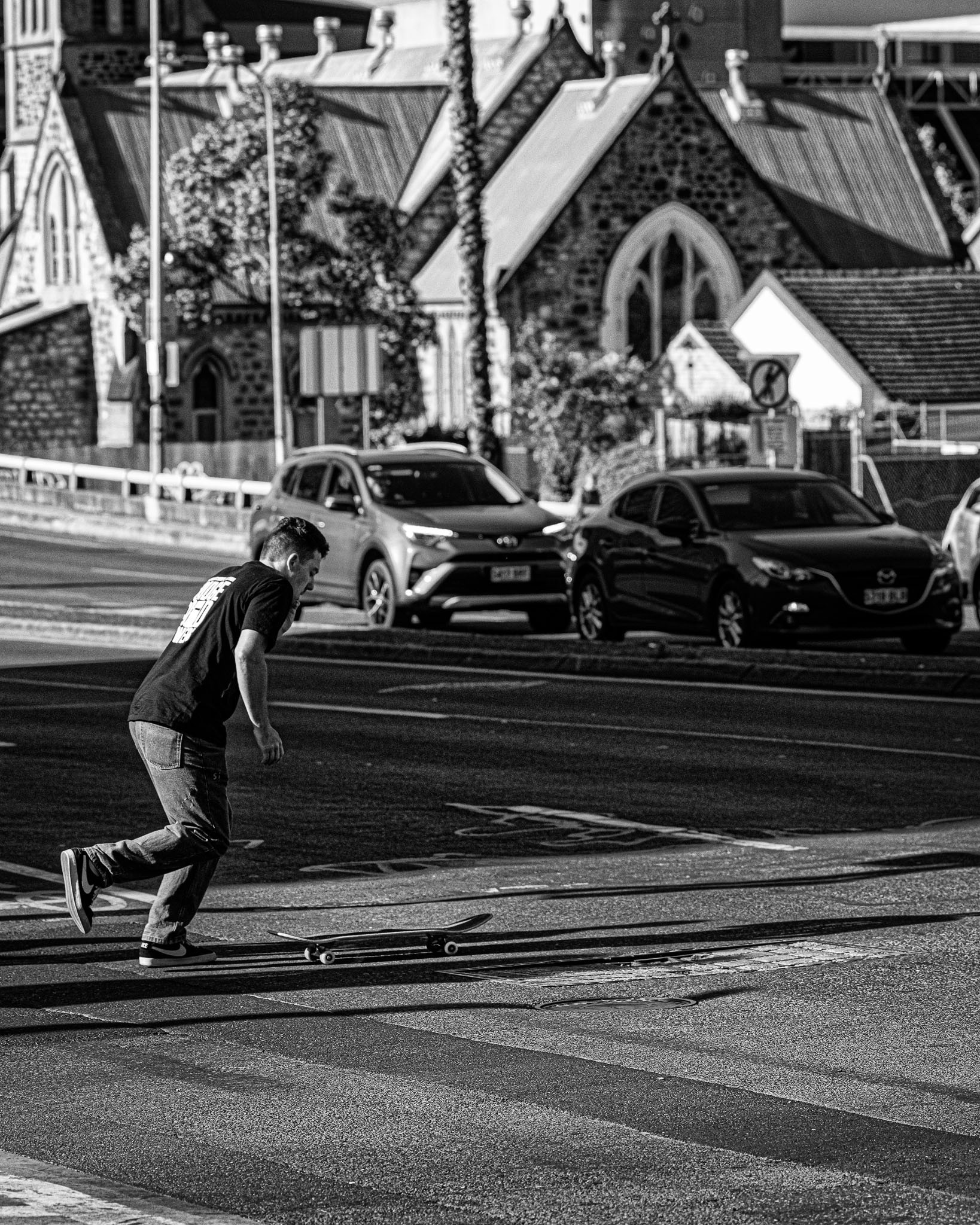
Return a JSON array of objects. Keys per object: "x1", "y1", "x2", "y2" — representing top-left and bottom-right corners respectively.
[{"x1": 266, "y1": 913, "x2": 494, "y2": 965}]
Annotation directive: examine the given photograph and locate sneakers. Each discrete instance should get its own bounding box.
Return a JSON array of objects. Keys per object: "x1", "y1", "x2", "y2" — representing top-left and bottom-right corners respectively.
[
  {"x1": 63, "y1": 847, "x2": 102, "y2": 935},
  {"x1": 139, "y1": 936, "x2": 219, "y2": 969}
]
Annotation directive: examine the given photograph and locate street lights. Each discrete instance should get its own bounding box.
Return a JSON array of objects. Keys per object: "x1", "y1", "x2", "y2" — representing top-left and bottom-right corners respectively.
[{"x1": 158, "y1": 51, "x2": 297, "y2": 484}]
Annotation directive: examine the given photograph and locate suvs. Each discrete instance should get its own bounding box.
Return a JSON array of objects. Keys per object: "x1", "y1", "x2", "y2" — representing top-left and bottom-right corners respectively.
[{"x1": 247, "y1": 439, "x2": 577, "y2": 638}]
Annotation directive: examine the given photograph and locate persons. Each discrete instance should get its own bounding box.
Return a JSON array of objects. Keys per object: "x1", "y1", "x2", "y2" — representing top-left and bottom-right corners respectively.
[
  {"x1": 422, "y1": 466, "x2": 468, "y2": 504},
  {"x1": 61, "y1": 518, "x2": 330, "y2": 967},
  {"x1": 366, "y1": 470, "x2": 405, "y2": 502}
]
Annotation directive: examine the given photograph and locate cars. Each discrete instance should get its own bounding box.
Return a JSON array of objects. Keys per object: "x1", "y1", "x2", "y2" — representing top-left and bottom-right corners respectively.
[
  {"x1": 572, "y1": 462, "x2": 964, "y2": 650},
  {"x1": 938, "y1": 476, "x2": 980, "y2": 623}
]
